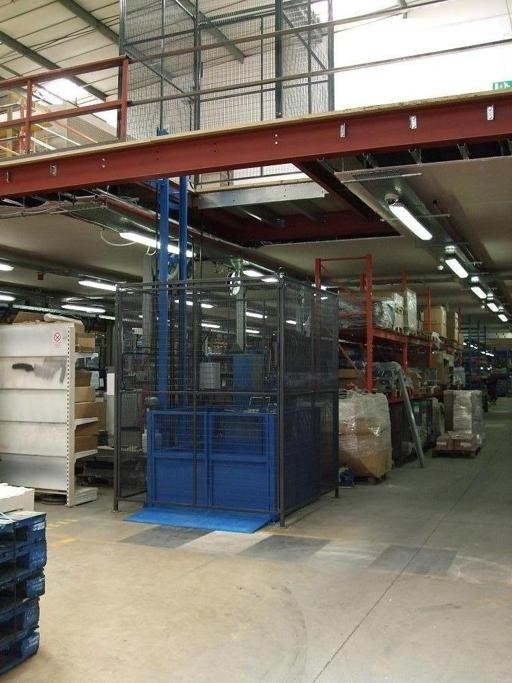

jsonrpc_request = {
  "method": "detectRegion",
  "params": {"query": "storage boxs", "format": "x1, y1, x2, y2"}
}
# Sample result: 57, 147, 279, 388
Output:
72, 330, 99, 452
449, 314, 460, 326
420, 305, 445, 322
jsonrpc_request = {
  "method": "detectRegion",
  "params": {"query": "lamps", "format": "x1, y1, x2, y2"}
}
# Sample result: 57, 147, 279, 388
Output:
118, 228, 198, 259
0, 260, 312, 343
469, 284, 487, 299
487, 302, 507, 324
386, 195, 434, 243
442, 253, 470, 279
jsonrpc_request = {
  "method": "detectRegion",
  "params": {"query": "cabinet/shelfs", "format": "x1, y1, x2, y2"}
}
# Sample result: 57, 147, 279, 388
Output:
318, 319, 512, 476
0, 318, 100, 508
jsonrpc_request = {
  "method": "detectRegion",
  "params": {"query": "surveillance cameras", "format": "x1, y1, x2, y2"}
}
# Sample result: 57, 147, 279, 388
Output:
385, 194, 399, 205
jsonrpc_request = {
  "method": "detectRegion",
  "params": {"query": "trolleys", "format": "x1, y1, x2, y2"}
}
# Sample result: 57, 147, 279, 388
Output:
354, 459, 395, 484
433, 390, 487, 457
